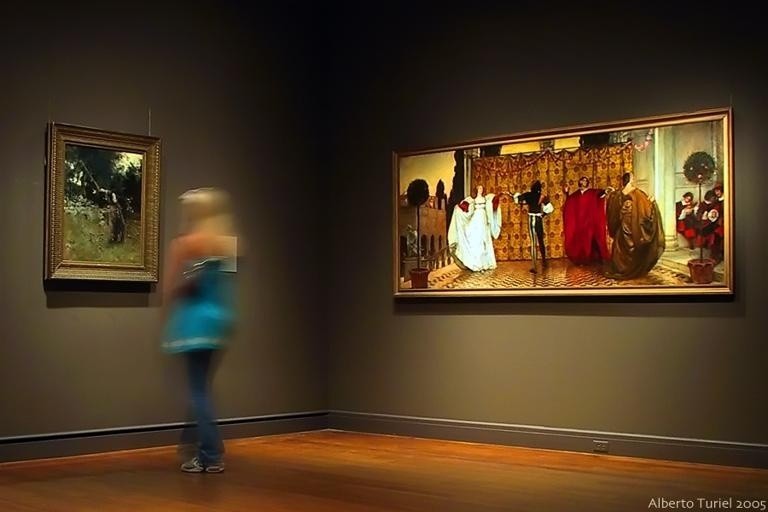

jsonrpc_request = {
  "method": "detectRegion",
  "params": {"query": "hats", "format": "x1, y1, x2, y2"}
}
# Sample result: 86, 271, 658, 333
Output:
712, 183, 723, 191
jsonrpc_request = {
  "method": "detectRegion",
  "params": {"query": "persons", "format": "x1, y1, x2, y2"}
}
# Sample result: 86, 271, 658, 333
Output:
109, 192, 124, 240
560, 176, 613, 266
507, 180, 554, 275
448, 184, 502, 273
704, 185, 724, 260
694, 191, 720, 246
151, 183, 243, 476
675, 192, 700, 252
602, 171, 666, 280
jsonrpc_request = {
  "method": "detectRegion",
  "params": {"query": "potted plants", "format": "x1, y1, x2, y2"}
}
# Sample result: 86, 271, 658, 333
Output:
683, 152, 720, 285
405, 177, 468, 289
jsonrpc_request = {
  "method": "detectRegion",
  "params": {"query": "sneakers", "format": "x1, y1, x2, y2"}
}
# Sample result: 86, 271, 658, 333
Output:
180, 455, 226, 474
528, 267, 538, 274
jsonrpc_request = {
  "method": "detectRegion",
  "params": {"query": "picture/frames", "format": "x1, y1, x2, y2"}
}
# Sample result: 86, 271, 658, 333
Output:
43, 119, 163, 285
391, 106, 737, 298
